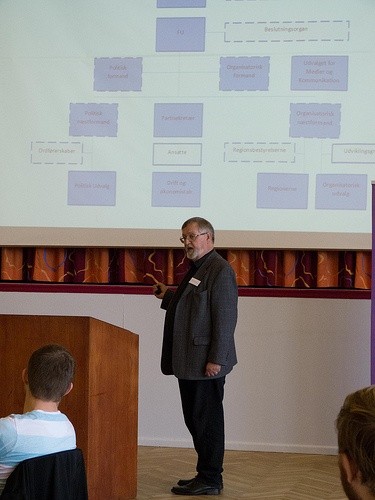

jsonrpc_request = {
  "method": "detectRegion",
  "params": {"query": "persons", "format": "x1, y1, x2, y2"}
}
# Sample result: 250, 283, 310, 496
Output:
153, 216, 238, 496
0, 344, 76, 500
336, 384, 375, 500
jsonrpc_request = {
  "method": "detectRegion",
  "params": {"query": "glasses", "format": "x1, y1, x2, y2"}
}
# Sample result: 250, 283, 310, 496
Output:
179, 232, 210, 243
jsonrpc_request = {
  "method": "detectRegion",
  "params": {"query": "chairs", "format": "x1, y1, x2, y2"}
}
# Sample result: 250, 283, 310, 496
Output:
0, 448, 89, 500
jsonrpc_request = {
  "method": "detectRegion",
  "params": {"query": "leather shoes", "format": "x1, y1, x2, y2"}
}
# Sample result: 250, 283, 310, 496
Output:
171, 477, 221, 494
177, 475, 224, 489
